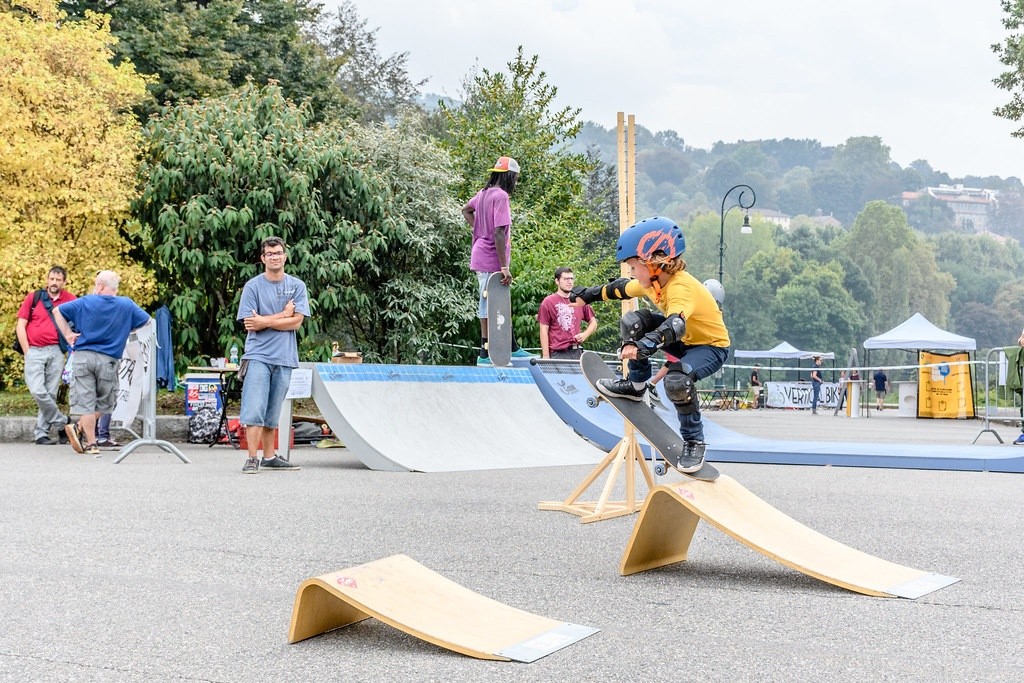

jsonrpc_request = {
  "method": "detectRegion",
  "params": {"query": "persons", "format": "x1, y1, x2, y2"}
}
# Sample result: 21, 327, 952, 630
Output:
751, 363, 762, 408
850, 370, 862, 390
16, 266, 77, 444
1013, 330, 1024, 445
838, 370, 848, 410
873, 370, 887, 410
810, 358, 823, 414
461, 156, 540, 367
568, 216, 730, 474
536, 267, 597, 359
236, 236, 311, 473
210, 385, 216, 393
52, 271, 151, 454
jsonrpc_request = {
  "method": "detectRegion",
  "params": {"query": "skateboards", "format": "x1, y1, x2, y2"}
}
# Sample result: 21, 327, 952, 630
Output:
579, 351, 722, 482
482, 272, 513, 367
292, 414, 329, 430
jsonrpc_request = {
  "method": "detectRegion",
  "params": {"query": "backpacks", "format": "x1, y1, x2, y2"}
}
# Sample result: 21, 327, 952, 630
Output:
187, 401, 227, 444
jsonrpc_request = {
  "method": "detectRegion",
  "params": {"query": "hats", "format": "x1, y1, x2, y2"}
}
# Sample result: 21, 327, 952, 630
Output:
486, 156, 520, 173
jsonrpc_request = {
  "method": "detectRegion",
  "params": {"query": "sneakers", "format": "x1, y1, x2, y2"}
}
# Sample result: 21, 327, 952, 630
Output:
596, 373, 647, 402
510, 347, 540, 359
260, 454, 301, 470
242, 457, 259, 474
1013, 433, 1024, 445
477, 355, 513, 367
677, 440, 710, 473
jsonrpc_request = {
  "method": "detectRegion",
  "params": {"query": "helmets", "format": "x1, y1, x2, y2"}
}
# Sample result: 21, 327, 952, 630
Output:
616, 216, 686, 263
702, 279, 726, 305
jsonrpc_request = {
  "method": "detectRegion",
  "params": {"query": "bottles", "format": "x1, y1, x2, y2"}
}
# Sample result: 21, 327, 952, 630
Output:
230, 345, 238, 363
737, 381, 740, 389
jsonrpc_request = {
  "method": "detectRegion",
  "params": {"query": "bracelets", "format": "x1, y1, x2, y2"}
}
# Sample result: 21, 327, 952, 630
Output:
501, 267, 507, 270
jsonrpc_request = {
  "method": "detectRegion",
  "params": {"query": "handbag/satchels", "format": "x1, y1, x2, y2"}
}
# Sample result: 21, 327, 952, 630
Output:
13, 339, 24, 355
57, 334, 69, 354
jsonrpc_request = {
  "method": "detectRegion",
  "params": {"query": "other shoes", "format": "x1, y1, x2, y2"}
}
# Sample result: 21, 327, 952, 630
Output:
96, 440, 120, 451
58, 416, 71, 444
83, 443, 99, 454
648, 382, 660, 401
64, 421, 85, 453
36, 436, 57, 445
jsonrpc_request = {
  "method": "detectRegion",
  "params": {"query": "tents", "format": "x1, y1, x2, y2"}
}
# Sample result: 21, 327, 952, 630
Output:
862, 312, 978, 420
733, 341, 836, 409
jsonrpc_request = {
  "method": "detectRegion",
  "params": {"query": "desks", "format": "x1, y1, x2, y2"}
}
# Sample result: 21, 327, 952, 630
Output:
716, 390, 751, 410
892, 380, 918, 418
695, 389, 717, 411
844, 381, 869, 417
188, 365, 242, 450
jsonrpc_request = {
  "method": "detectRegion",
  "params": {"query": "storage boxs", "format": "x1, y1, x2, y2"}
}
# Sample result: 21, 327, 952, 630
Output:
236, 425, 295, 450
218, 415, 240, 442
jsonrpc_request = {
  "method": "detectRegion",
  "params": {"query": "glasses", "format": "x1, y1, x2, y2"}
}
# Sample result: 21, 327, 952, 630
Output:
263, 251, 285, 258
